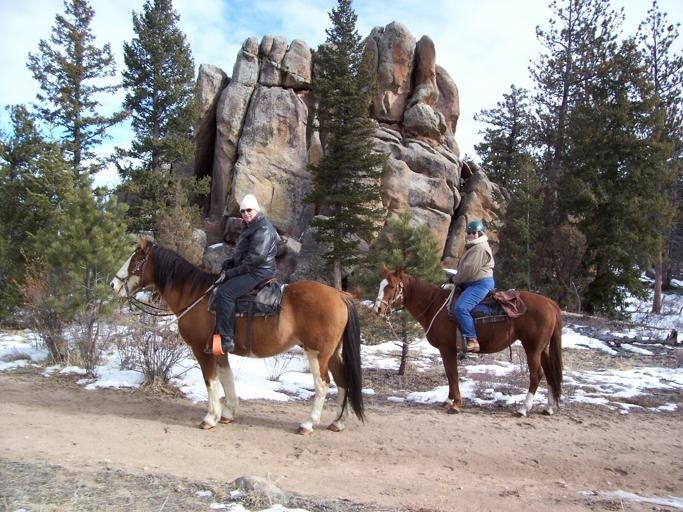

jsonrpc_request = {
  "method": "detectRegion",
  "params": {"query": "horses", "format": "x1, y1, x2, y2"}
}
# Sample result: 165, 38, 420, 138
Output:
109, 233, 368, 436
372, 261, 564, 417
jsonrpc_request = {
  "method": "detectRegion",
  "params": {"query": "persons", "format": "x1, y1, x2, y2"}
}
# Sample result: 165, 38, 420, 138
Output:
202, 191, 284, 355
442, 218, 498, 355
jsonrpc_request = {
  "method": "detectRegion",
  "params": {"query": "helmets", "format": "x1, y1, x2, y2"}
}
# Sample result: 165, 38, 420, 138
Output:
463, 220, 484, 233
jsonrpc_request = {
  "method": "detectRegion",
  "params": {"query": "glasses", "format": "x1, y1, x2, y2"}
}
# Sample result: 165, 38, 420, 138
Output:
466, 231, 477, 236
240, 208, 253, 213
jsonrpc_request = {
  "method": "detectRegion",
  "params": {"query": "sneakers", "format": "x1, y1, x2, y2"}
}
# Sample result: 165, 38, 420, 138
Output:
202, 337, 234, 354
463, 338, 481, 354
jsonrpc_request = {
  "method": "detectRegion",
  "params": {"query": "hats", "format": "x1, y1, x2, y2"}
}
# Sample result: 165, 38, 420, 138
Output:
238, 193, 261, 213
